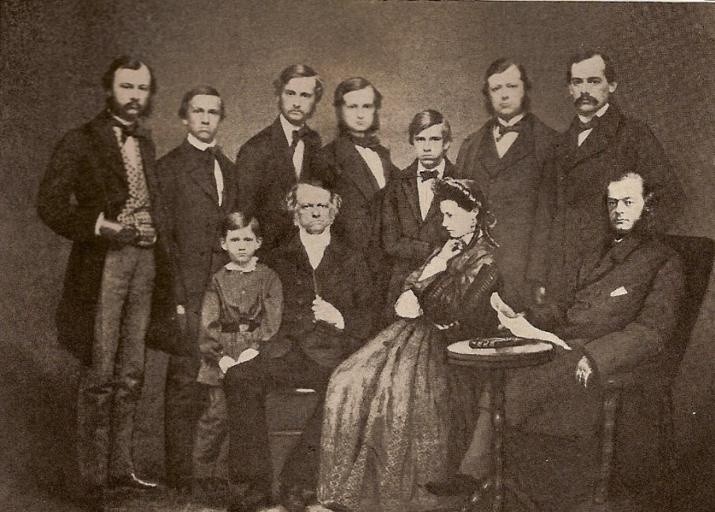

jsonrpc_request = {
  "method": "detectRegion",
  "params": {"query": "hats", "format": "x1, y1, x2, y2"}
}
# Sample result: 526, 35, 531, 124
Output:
431, 176, 490, 216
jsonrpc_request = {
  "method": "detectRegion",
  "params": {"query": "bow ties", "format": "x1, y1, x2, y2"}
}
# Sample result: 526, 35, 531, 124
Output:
496, 123, 526, 134
293, 126, 311, 141
200, 145, 226, 171
356, 135, 381, 150
117, 124, 147, 144
576, 114, 601, 130
420, 167, 441, 181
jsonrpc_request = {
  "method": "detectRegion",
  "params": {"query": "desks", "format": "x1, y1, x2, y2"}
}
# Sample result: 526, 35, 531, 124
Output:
447, 339, 554, 512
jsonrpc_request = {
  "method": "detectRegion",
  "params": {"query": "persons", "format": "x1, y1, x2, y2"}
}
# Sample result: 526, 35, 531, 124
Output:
150, 85, 239, 459
382, 109, 471, 329
36, 56, 161, 512
425, 167, 685, 512
231, 63, 326, 269
322, 76, 403, 328
305, 177, 509, 512
190, 211, 285, 499
524, 49, 688, 315
227, 167, 375, 511
455, 56, 561, 314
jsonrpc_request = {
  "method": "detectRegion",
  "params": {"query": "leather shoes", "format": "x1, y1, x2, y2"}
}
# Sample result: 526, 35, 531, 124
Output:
112, 470, 163, 494
226, 482, 273, 511
275, 475, 312, 512
79, 474, 114, 512
422, 473, 484, 498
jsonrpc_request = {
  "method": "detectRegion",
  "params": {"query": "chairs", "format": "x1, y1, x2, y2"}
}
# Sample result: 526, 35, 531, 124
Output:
261, 217, 371, 437
521, 232, 715, 511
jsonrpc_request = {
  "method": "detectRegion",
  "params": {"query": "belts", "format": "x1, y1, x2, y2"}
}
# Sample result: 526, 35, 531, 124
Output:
222, 320, 266, 335
